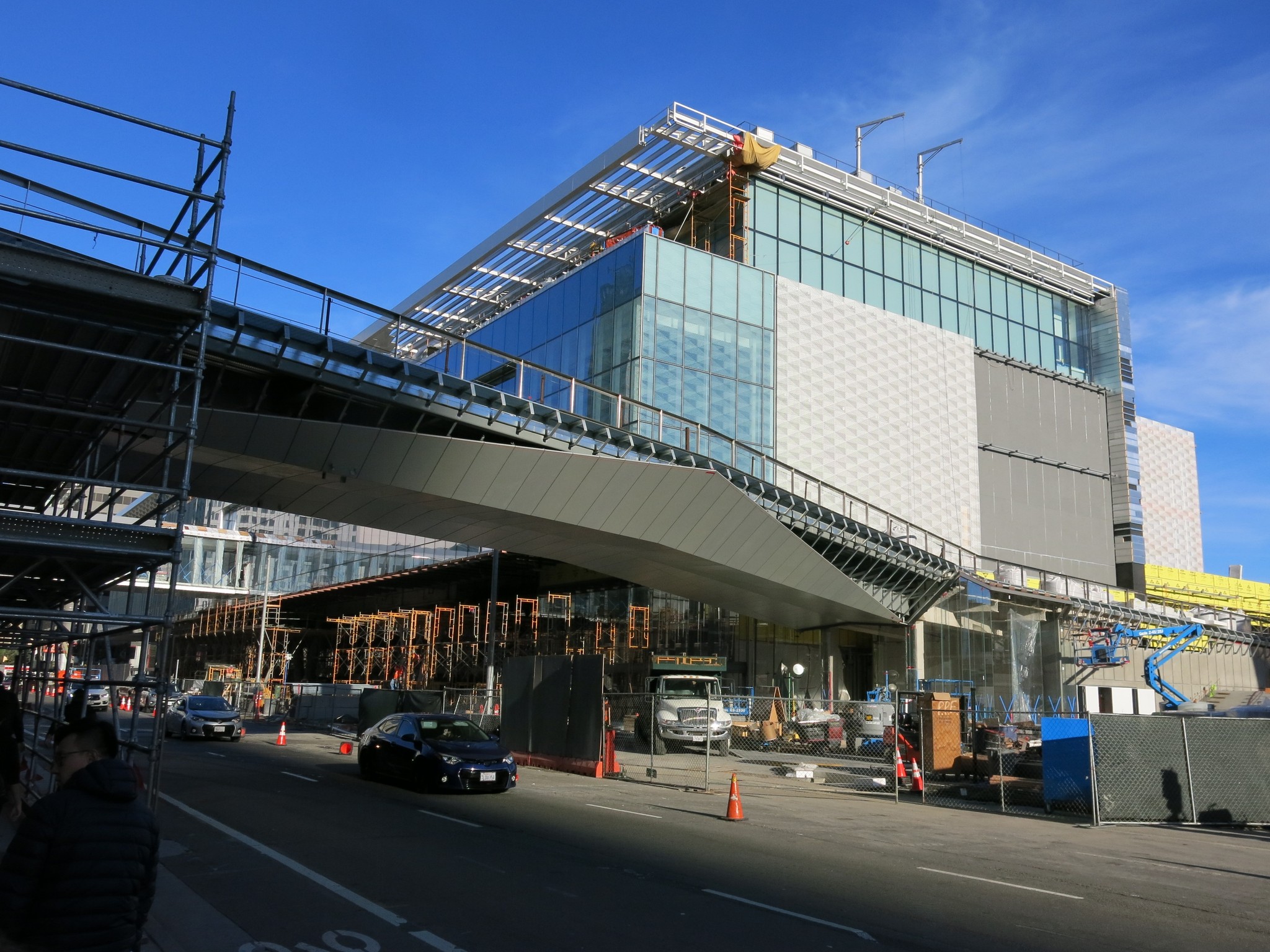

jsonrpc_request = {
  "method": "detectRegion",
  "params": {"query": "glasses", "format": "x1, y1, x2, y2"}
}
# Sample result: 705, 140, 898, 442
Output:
55, 750, 94, 768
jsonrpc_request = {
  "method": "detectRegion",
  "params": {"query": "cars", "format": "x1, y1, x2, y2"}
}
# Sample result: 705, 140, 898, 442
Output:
356, 712, 518, 796
165, 695, 243, 743
0, 665, 110, 712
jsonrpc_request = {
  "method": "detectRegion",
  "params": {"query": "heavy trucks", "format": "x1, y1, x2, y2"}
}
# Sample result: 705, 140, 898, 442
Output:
634, 651, 733, 757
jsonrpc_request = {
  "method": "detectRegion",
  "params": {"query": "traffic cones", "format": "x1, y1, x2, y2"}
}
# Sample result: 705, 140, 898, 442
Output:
119, 696, 126, 710
273, 721, 287, 746
718, 774, 749, 821
893, 746, 907, 778
909, 759, 928, 792
338, 741, 354, 755
124, 697, 132, 711
151, 705, 156, 717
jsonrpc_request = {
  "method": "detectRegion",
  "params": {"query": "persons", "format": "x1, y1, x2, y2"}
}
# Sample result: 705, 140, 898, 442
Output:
0, 718, 159, 952
226, 665, 234, 678
390, 652, 421, 690
0, 671, 25, 822
253, 685, 263, 714
44, 689, 97, 751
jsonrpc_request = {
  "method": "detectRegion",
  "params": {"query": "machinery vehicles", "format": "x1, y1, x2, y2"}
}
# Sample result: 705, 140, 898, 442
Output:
1113, 620, 1270, 720
